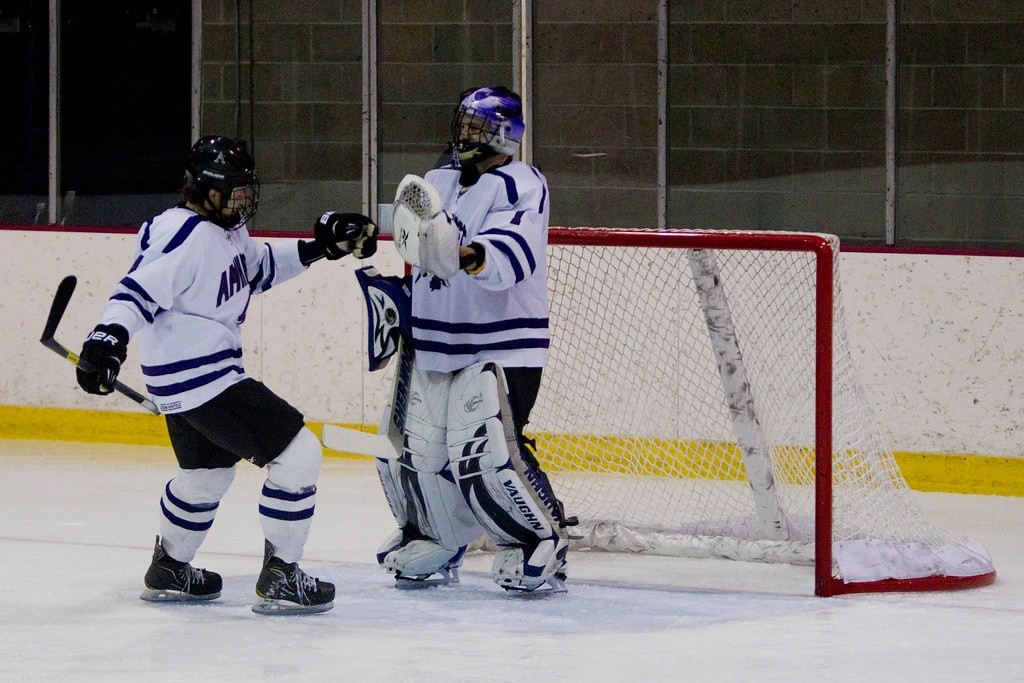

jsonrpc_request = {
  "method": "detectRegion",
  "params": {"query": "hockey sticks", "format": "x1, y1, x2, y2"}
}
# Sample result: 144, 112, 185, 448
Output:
319, 261, 413, 460
39, 275, 163, 416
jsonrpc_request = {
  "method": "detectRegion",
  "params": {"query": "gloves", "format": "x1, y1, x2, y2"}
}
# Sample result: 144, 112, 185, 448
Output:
76, 323, 129, 395
314, 212, 379, 260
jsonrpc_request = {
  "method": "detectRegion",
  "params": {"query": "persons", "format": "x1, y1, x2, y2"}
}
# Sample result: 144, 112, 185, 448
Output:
375, 86, 568, 589
76, 134, 380, 605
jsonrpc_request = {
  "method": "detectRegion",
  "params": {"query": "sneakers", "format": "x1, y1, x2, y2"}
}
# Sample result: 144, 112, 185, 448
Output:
140, 534, 223, 602
499, 559, 568, 600
251, 538, 336, 615
394, 544, 468, 589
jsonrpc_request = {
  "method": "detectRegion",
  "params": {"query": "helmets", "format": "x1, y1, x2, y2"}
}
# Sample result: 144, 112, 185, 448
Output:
185, 135, 260, 232
450, 86, 525, 170
451, 88, 481, 111
